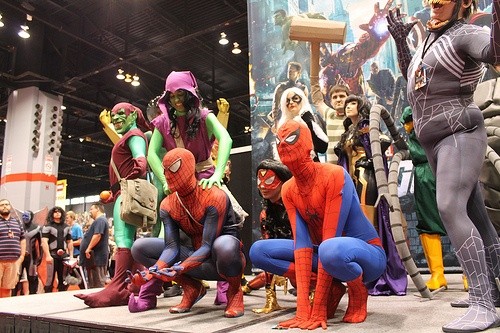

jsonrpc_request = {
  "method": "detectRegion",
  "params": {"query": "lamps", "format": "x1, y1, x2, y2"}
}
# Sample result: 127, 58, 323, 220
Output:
231, 41, 242, 55
16, 19, 32, 40
116, 65, 125, 80
125, 67, 132, 83
131, 69, 141, 87
219, 30, 230, 46
0, 11, 5, 28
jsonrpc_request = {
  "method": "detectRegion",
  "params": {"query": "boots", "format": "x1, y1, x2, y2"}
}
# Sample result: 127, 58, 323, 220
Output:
419, 231, 450, 292
462, 273, 470, 292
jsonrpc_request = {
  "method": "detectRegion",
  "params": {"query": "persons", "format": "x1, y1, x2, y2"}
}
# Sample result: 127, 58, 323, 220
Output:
387, 1, 500, 332
0, 198, 116, 298
370, 97, 499, 302
247, 120, 387, 330
334, 94, 408, 296
273, 86, 329, 162
243, 158, 315, 315
73, 69, 248, 318
289, 18, 350, 165
248, 1, 397, 121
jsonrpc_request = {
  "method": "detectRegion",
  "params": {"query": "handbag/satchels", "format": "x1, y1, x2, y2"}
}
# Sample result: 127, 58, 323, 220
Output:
119, 173, 159, 232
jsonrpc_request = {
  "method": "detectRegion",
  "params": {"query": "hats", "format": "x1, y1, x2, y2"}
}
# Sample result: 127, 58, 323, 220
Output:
157, 70, 205, 106
